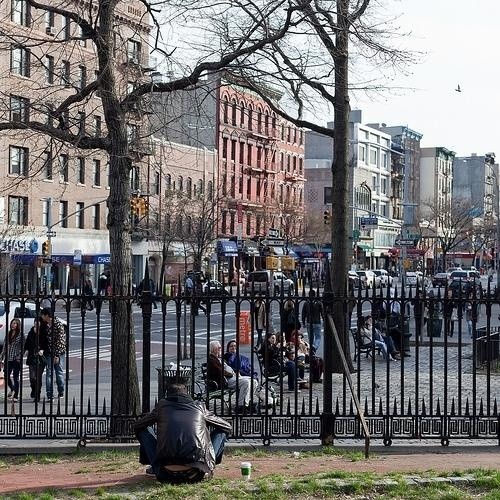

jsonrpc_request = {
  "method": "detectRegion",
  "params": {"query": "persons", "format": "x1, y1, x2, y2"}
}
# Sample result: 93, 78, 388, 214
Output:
131, 278, 157, 309
83, 269, 112, 312
0, 318, 26, 402
183, 273, 207, 316
223, 340, 258, 381
38, 306, 65, 402
133, 383, 233, 484
209, 341, 258, 414
350, 261, 493, 360
25, 316, 46, 402
253, 268, 325, 392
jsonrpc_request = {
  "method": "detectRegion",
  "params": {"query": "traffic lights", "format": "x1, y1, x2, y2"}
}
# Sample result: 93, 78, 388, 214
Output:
140, 198, 150, 217
323, 206, 332, 228
131, 198, 138, 215
41, 239, 52, 258
262, 256, 279, 270
280, 257, 299, 272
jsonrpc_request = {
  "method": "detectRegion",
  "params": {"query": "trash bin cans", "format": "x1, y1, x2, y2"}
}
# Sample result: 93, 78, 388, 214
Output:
156, 365, 194, 407
424, 316, 443, 337
386, 315, 410, 352
166, 284, 179, 298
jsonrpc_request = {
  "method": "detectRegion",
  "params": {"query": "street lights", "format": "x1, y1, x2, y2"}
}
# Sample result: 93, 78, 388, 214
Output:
460, 157, 500, 291
349, 139, 409, 299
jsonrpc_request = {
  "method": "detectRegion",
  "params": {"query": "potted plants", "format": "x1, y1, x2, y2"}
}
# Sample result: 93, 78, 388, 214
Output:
425, 294, 443, 338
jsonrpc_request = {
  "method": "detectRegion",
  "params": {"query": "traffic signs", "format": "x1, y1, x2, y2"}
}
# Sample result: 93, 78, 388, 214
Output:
259, 237, 284, 247
269, 228, 280, 238
45, 231, 56, 238
395, 238, 415, 247
359, 216, 378, 230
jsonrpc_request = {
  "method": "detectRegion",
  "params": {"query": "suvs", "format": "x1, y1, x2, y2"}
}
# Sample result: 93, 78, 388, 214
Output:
243, 270, 296, 298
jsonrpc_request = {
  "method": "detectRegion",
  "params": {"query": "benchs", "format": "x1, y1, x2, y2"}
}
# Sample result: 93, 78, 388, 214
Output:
254, 338, 311, 391
197, 360, 239, 415
349, 327, 385, 362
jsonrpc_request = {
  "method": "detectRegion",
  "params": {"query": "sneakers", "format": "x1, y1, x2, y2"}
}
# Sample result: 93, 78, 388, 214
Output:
146, 466, 156, 476
235, 402, 257, 414
8, 390, 65, 402
448, 332, 454, 337
287, 377, 324, 393
387, 351, 411, 361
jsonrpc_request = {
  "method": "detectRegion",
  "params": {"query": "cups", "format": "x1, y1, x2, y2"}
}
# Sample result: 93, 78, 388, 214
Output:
240, 462, 252, 479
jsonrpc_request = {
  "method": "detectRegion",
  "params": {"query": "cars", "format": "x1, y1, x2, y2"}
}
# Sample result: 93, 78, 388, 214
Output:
0, 300, 70, 353
347, 264, 484, 292
186, 269, 231, 303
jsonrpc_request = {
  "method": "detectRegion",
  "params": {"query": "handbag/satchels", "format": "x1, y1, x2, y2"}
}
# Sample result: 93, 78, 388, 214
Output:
451, 308, 459, 321
0, 367, 5, 386
